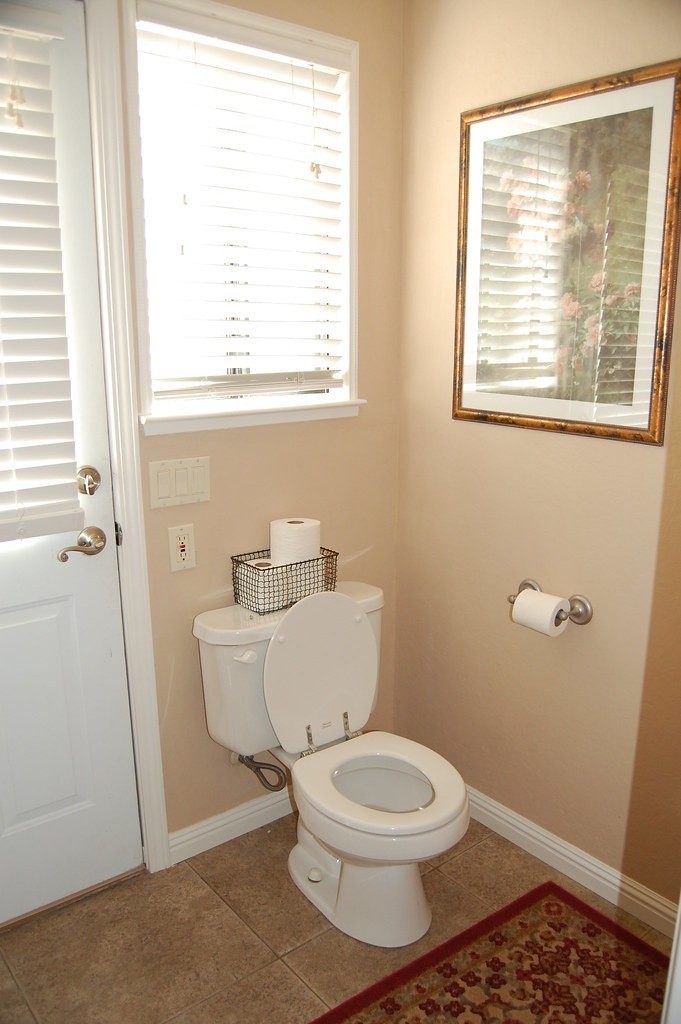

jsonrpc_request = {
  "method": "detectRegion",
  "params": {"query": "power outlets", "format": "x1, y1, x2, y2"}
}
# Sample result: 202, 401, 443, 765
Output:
168, 524, 196, 573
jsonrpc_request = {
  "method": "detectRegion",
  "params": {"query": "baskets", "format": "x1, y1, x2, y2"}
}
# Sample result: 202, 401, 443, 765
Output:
232, 545, 339, 614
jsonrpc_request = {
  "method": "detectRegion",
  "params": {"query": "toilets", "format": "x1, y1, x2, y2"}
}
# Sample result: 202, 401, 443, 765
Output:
191, 572, 471, 951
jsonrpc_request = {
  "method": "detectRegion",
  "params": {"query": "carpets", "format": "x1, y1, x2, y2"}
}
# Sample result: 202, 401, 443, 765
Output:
307, 880, 670, 1024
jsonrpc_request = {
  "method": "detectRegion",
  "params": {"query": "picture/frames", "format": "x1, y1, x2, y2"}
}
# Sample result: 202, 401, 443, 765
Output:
450, 57, 681, 448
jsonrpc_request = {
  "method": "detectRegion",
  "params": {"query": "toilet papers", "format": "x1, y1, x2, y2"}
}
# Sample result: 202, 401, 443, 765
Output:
511, 588, 571, 638
235, 516, 327, 614
